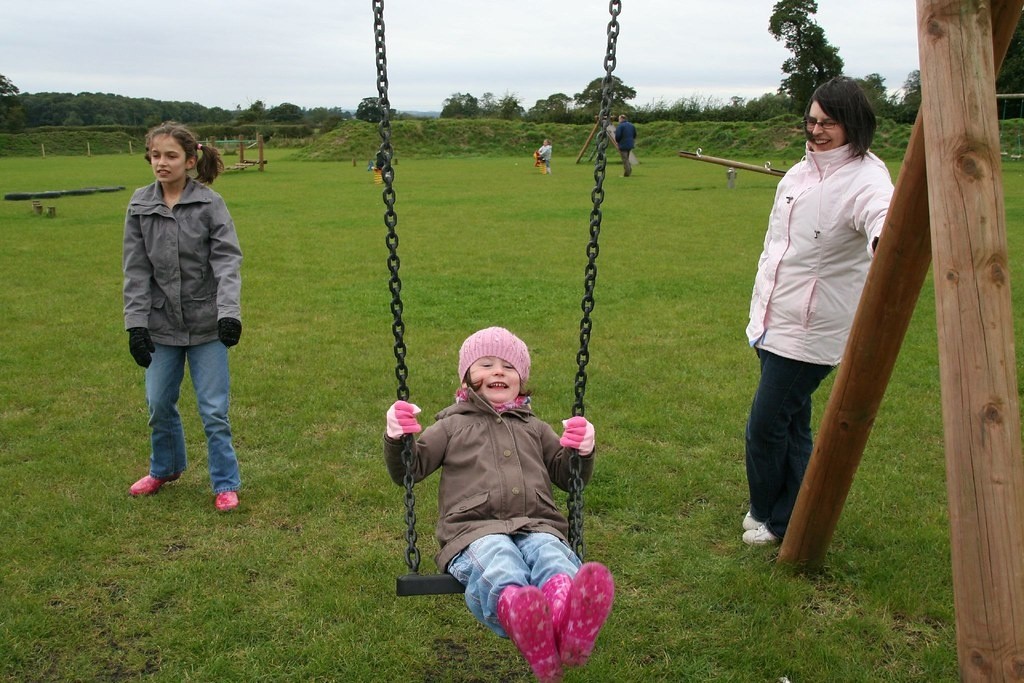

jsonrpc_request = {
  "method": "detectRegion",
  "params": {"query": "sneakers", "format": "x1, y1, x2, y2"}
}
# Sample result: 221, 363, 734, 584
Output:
743, 509, 765, 530
742, 522, 780, 545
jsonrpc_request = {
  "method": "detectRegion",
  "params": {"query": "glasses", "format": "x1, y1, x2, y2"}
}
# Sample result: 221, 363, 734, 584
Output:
801, 117, 839, 129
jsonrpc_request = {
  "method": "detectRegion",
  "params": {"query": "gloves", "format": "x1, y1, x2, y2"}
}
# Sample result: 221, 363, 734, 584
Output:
560, 416, 595, 456
387, 400, 422, 440
217, 317, 242, 347
129, 326, 155, 368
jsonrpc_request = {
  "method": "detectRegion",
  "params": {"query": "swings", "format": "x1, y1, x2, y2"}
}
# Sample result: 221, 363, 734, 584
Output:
371, 0, 623, 597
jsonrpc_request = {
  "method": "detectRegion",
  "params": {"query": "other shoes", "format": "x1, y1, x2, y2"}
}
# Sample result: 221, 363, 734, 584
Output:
129, 469, 181, 497
215, 492, 238, 511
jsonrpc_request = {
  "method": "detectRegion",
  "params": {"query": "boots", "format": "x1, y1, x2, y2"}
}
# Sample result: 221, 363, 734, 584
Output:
542, 561, 614, 666
497, 583, 564, 683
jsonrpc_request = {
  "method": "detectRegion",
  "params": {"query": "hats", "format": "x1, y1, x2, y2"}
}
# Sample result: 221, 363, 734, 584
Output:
458, 327, 531, 387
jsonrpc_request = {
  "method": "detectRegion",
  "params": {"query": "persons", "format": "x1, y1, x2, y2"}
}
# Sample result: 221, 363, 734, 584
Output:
742, 77, 895, 544
383, 327, 615, 683
538, 139, 552, 174
615, 114, 637, 177
122, 125, 243, 511
374, 149, 389, 184
